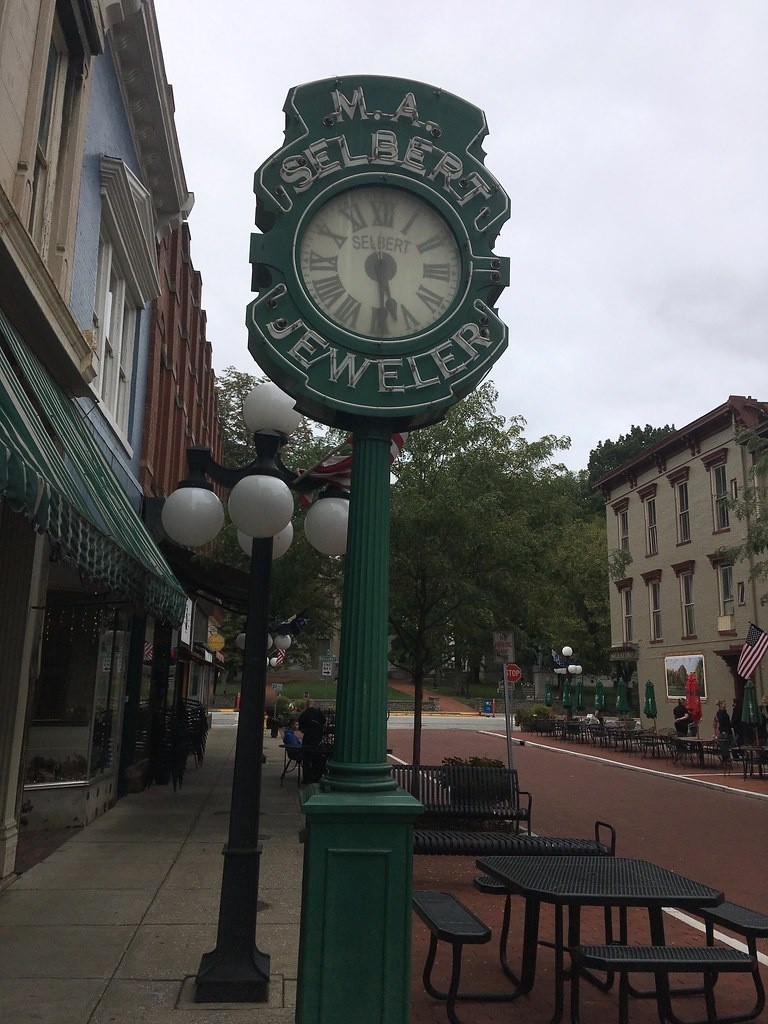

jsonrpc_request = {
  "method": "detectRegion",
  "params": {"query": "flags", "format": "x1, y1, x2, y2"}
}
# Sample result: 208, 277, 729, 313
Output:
276, 649, 285, 666
296, 431, 408, 506
275, 612, 309, 635
549, 647, 566, 674
736, 623, 768, 679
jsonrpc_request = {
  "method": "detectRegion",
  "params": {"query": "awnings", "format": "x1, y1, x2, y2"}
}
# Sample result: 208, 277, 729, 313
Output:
0, 308, 189, 631
163, 542, 277, 621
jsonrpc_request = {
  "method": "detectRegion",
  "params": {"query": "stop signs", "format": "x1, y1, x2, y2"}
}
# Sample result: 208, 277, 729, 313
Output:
505, 664, 521, 682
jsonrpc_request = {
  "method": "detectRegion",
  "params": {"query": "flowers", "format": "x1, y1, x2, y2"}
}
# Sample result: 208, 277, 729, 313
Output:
319, 734, 335, 745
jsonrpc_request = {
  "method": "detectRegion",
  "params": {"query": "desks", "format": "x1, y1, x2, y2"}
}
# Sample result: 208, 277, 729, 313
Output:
476, 857, 726, 1017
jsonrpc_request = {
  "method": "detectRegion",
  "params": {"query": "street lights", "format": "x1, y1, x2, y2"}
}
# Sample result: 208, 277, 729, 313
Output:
154, 381, 349, 1007
561, 646, 583, 720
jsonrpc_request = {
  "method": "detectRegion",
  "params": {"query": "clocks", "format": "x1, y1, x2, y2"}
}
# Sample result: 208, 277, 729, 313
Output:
249, 74, 512, 430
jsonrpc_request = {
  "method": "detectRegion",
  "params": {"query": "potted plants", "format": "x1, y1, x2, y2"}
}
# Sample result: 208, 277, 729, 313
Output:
444, 757, 507, 806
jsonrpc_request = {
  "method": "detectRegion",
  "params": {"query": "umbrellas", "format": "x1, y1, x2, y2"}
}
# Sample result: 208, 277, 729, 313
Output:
615, 680, 631, 728
643, 681, 657, 728
544, 683, 552, 707
684, 674, 702, 739
741, 681, 762, 745
563, 681, 607, 722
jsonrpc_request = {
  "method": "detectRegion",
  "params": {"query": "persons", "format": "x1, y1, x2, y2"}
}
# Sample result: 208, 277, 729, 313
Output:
758, 696, 768, 762
715, 699, 732, 762
673, 698, 698, 753
298, 699, 327, 787
586, 710, 603, 724
284, 719, 303, 767
730, 698, 745, 757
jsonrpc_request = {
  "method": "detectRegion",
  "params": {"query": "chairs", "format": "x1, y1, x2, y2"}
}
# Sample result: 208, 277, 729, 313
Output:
154, 698, 209, 768
278, 744, 302, 779
536, 721, 767, 780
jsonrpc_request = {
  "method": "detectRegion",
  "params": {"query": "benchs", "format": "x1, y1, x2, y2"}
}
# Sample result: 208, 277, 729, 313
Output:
393, 765, 532, 835
570, 945, 753, 1024
701, 901, 768, 947
414, 821, 615, 856
411, 890, 491, 1024
476, 876, 517, 895
